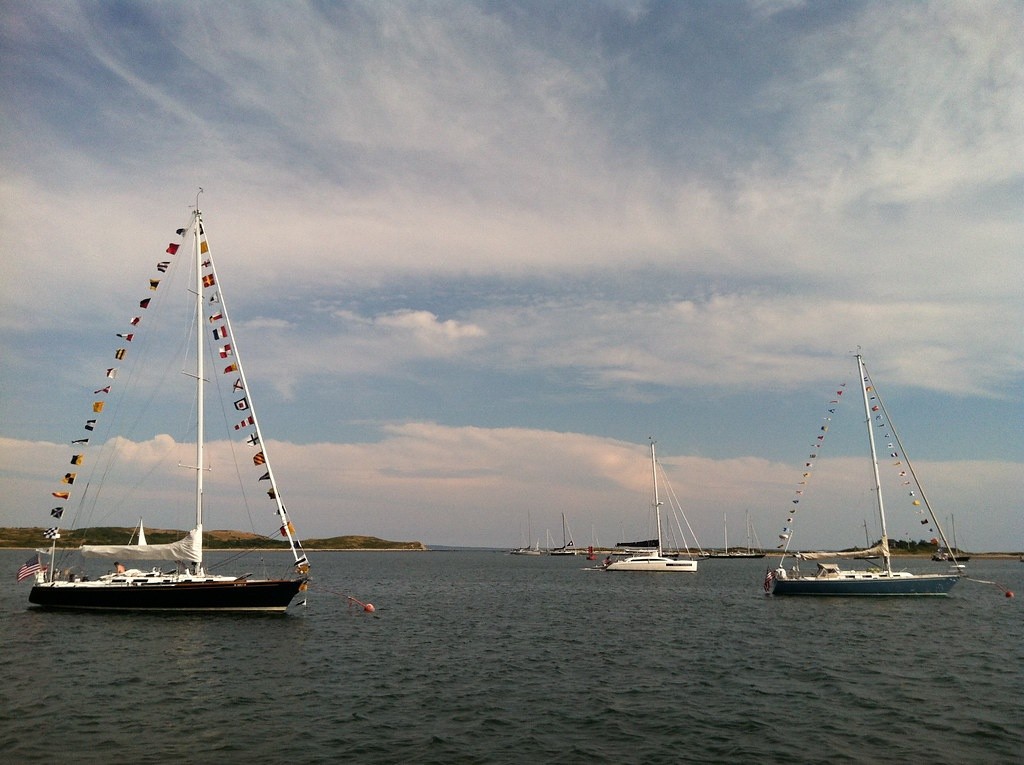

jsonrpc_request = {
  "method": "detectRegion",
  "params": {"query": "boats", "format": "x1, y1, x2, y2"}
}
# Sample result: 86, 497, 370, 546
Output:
698, 551, 710, 556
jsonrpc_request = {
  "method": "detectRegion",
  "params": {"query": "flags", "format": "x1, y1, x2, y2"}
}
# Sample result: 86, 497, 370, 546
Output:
35, 507, 65, 555
885, 434, 910, 486
910, 491, 938, 545
113, 298, 151, 360
812, 383, 846, 447
234, 396, 271, 481
764, 569, 774, 592
775, 453, 815, 549
267, 487, 308, 567
149, 228, 188, 290
16, 554, 42, 582
864, 377, 885, 428
209, 308, 243, 393
85, 367, 118, 432
51, 438, 89, 501
194, 225, 219, 305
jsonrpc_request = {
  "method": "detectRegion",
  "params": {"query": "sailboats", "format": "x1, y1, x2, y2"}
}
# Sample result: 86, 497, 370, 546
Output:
710, 509, 765, 558
510, 506, 541, 556
765, 353, 967, 596
660, 515, 679, 556
580, 522, 601, 554
854, 520, 880, 559
540, 513, 578, 556
611, 520, 633, 555
596, 438, 710, 573
932, 512, 971, 561
29, 207, 313, 618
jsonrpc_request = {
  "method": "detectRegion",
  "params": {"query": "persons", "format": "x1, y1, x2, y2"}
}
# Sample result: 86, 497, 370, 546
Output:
114, 562, 125, 573
